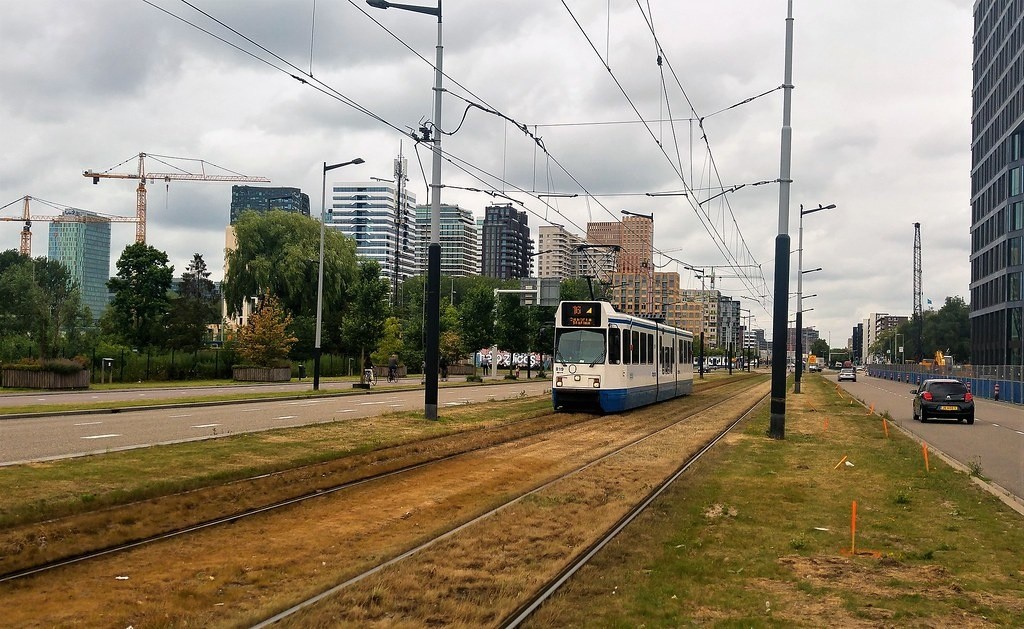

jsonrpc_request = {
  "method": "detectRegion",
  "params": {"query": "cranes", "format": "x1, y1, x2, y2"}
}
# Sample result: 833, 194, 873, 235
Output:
82, 151, 272, 245
0, 192, 141, 258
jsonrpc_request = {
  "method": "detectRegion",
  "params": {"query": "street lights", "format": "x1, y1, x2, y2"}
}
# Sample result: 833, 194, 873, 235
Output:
737, 308, 751, 372
794, 204, 837, 394
620, 209, 654, 314
314, 158, 366, 390
365, 0, 445, 422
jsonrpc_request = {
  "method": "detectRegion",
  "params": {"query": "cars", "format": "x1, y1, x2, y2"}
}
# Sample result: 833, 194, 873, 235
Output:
910, 378, 975, 425
835, 360, 868, 371
698, 364, 711, 373
837, 367, 856, 382
731, 357, 772, 369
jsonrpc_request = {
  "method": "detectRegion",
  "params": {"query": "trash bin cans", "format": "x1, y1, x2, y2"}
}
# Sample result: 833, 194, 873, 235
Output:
103, 358, 113, 371
299, 365, 306, 378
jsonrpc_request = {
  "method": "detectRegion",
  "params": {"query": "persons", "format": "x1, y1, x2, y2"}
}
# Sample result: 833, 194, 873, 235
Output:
481, 355, 490, 377
515, 365, 520, 379
388, 355, 399, 382
363, 356, 375, 383
439, 355, 450, 382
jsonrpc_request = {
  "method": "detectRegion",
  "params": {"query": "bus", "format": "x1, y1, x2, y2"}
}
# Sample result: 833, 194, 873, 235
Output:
550, 243, 695, 416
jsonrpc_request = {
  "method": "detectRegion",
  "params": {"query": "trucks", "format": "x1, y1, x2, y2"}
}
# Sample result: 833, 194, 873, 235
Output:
791, 357, 825, 373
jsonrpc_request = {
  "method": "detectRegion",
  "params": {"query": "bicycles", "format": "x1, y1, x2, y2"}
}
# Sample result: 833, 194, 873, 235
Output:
387, 364, 399, 383
364, 366, 377, 386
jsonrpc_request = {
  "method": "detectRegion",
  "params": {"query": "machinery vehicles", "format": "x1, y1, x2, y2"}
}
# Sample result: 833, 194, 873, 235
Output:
920, 350, 953, 376
905, 222, 923, 364
